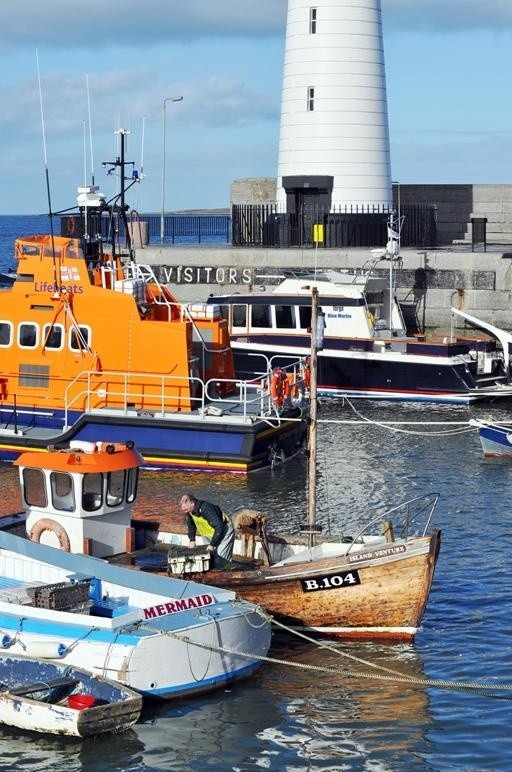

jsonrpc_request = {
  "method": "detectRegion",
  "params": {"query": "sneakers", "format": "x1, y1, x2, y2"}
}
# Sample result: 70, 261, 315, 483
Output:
210, 567, 223, 572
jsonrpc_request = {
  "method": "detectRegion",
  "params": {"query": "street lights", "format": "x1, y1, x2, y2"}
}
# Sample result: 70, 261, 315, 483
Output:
160, 95, 184, 244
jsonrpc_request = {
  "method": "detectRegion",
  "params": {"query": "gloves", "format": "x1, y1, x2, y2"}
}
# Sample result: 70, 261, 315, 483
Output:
187, 541, 196, 549
206, 544, 214, 552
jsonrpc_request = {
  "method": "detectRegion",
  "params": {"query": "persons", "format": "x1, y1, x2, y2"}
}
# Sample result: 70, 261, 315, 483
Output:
179, 495, 235, 570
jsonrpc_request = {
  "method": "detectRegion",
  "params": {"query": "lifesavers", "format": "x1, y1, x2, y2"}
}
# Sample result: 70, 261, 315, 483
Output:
304, 356, 311, 389
28, 518, 70, 552
271, 370, 290, 405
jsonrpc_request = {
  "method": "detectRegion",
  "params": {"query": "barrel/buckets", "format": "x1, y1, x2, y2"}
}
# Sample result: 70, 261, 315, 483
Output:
69, 694, 96, 711
